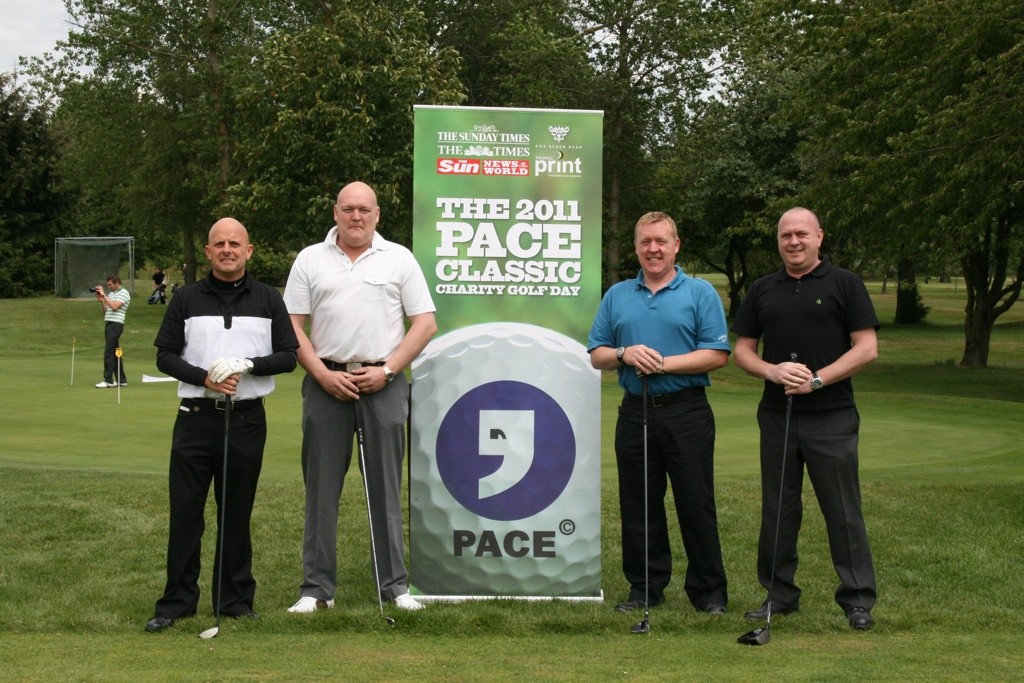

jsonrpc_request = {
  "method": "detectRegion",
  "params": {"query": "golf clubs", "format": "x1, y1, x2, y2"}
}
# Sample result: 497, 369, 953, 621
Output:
736, 352, 799, 645
353, 398, 397, 627
630, 379, 650, 634
197, 394, 231, 640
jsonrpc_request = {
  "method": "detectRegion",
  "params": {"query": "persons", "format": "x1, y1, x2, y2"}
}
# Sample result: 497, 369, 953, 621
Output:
282, 181, 438, 612
145, 218, 300, 633
151, 267, 168, 304
728, 207, 882, 629
95, 274, 130, 388
586, 213, 732, 615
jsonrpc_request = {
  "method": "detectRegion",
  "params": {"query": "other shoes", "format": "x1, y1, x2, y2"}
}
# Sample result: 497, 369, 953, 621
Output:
114, 381, 127, 386
704, 601, 726, 615
95, 381, 117, 387
616, 600, 642, 613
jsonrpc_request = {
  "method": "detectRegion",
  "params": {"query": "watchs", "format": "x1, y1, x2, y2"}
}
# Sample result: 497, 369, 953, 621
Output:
245, 359, 254, 373
810, 372, 823, 391
616, 346, 626, 364
101, 294, 105, 298
383, 365, 395, 384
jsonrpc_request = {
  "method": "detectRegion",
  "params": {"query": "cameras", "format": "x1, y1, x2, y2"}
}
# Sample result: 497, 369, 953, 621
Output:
90, 287, 98, 292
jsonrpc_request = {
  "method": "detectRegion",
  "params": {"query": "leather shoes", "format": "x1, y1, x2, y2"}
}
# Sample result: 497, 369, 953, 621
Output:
845, 608, 873, 630
243, 610, 259, 619
144, 616, 174, 635
741, 601, 799, 619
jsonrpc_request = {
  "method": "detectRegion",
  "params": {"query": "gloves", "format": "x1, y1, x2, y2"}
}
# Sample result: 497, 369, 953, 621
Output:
207, 356, 255, 385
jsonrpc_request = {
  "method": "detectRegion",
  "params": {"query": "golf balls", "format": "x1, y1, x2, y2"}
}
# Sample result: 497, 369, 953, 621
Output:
411, 321, 602, 597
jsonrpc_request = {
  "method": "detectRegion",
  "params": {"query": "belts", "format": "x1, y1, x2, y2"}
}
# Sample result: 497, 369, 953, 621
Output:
624, 386, 706, 408
319, 358, 388, 375
193, 397, 263, 411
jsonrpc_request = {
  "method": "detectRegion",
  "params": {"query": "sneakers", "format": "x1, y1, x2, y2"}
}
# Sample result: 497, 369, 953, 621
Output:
394, 593, 428, 612
288, 595, 334, 615
